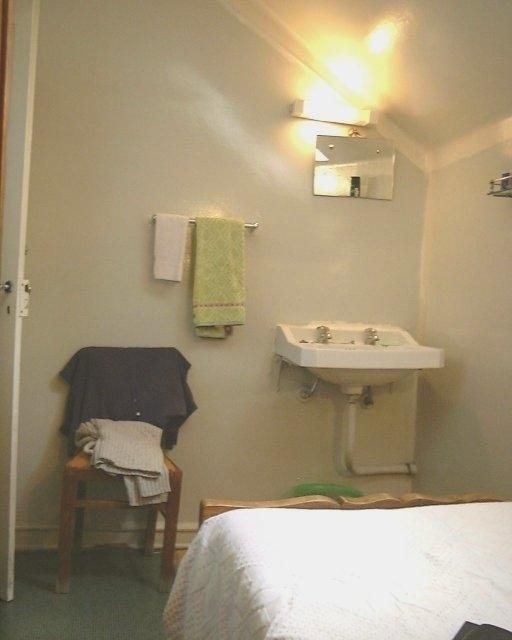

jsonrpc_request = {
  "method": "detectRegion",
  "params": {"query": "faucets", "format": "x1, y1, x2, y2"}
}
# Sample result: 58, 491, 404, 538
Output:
364, 328, 379, 345
316, 326, 332, 343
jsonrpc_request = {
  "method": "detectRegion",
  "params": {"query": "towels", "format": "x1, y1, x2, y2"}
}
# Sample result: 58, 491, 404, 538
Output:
191, 216, 247, 340
151, 212, 186, 282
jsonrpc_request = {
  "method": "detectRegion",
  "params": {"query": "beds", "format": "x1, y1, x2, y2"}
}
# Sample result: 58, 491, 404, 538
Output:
164, 488, 512, 640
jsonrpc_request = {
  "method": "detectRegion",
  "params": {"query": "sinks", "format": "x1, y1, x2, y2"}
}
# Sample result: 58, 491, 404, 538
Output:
272, 324, 446, 396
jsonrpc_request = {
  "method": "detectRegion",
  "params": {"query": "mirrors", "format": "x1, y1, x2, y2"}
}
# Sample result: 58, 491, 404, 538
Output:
311, 135, 398, 202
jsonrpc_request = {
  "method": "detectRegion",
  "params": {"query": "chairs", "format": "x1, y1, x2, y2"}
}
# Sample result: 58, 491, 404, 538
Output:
59, 345, 188, 596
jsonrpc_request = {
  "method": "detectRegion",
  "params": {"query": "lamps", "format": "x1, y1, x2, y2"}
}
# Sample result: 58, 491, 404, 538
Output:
288, 98, 377, 128
